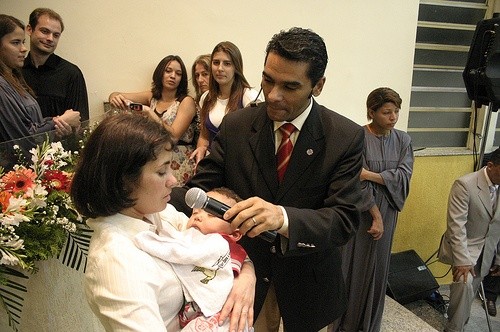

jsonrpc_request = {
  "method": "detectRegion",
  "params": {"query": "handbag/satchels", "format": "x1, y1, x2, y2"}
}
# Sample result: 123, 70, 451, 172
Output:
385, 250, 439, 305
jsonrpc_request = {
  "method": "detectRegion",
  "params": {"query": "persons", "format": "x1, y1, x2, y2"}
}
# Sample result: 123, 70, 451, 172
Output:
20, 8, 90, 152
132, 186, 255, 332
69, 110, 256, 332
0, 14, 81, 169
108, 41, 261, 188
437, 147, 500, 332
326, 87, 415, 332
167, 27, 365, 332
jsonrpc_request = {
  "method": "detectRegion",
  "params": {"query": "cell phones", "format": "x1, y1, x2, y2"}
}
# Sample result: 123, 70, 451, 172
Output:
126, 103, 143, 111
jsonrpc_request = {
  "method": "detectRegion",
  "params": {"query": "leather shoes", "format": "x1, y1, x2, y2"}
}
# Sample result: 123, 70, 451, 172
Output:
487, 300, 496, 316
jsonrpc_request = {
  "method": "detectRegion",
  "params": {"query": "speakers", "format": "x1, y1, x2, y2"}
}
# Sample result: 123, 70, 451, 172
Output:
386, 249, 440, 305
463, 16, 500, 112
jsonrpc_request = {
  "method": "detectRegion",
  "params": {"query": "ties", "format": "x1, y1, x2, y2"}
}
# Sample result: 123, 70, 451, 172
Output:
276, 123, 297, 188
490, 186, 497, 215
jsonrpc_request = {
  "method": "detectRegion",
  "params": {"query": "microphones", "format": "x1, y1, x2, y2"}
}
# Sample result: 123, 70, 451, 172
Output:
184, 187, 277, 243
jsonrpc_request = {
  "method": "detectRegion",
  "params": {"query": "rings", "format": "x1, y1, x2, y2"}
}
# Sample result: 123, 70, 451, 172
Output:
252, 217, 258, 225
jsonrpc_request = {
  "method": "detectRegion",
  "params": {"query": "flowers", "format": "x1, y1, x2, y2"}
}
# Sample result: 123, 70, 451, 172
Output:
0, 114, 104, 332
